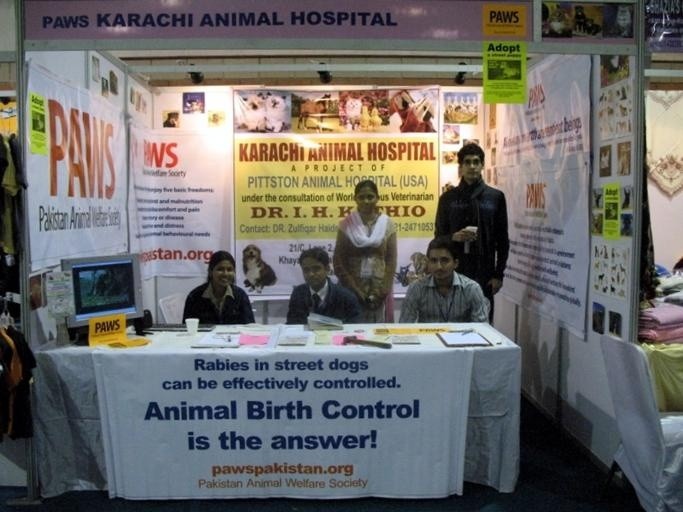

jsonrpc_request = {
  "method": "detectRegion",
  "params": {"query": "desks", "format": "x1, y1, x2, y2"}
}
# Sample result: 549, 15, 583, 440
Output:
31, 321, 520, 498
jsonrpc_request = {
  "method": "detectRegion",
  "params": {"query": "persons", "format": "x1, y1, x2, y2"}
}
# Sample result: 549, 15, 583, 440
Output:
350, 96, 382, 131
397, 236, 491, 323
433, 143, 510, 327
329, 180, 397, 323
180, 251, 255, 325
241, 91, 290, 132
29, 275, 41, 310
285, 246, 364, 324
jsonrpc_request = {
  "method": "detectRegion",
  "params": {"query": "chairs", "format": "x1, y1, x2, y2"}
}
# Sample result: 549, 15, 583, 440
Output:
600, 330, 683, 510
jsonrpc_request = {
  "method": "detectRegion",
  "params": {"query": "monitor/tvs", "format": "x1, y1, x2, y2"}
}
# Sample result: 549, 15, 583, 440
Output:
61, 253, 145, 346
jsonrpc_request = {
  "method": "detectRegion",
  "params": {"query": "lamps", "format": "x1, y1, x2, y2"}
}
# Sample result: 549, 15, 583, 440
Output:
455, 62, 466, 85
187, 63, 205, 85
316, 62, 334, 84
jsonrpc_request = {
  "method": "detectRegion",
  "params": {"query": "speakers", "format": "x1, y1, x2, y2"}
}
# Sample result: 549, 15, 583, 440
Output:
132, 309, 154, 336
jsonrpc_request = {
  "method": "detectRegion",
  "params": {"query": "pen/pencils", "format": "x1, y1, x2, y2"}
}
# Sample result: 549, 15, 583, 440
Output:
461, 330, 472, 336
229, 335, 231, 342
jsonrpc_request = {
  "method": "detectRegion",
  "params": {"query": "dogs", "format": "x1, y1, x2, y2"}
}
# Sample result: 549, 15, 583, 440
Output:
242, 91, 287, 134
343, 94, 366, 132
240, 244, 277, 293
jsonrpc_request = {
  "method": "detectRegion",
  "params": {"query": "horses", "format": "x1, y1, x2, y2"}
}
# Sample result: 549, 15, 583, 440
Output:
297, 94, 331, 133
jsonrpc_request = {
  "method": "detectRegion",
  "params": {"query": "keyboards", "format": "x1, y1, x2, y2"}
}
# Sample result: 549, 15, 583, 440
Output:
133, 323, 216, 332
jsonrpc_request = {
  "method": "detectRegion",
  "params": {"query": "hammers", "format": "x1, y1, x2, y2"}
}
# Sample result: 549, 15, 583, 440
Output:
344, 336, 392, 349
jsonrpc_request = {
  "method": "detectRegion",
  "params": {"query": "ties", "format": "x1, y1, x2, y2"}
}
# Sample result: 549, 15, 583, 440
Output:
310, 293, 322, 314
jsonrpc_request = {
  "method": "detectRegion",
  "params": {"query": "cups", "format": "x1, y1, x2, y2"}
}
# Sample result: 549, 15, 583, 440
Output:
467, 226, 477, 240
185, 318, 199, 334
